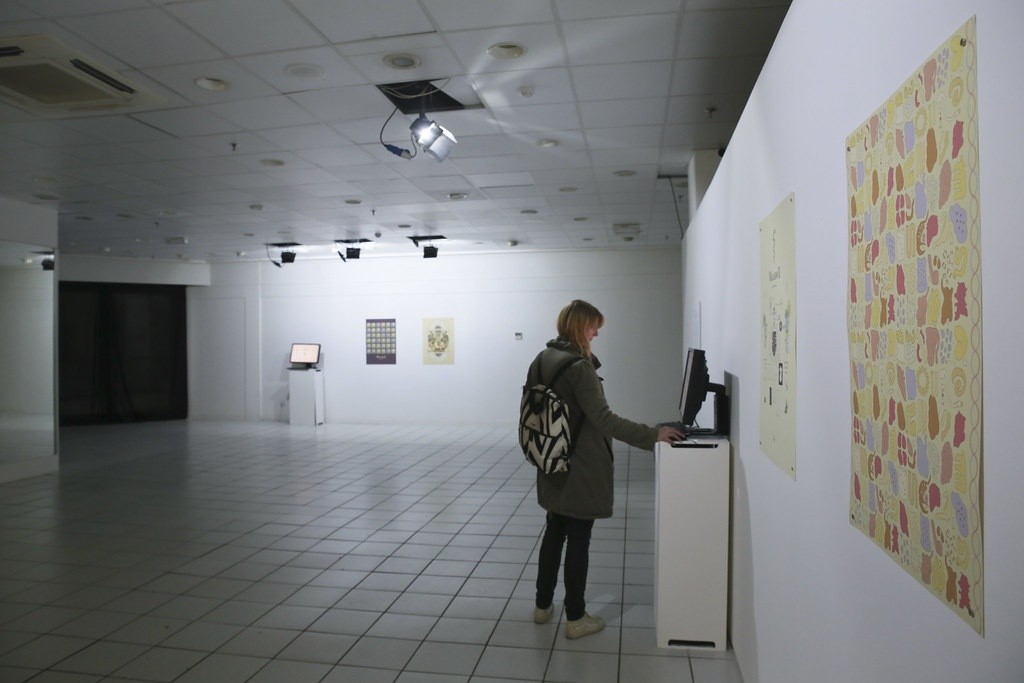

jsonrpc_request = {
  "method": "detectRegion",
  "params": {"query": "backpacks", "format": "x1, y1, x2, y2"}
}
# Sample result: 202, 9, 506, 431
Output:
519, 351, 586, 473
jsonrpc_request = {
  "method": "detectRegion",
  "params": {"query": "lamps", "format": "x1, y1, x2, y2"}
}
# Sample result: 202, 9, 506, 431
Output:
41, 252, 54, 270
410, 91, 458, 162
424, 239, 438, 258
346, 243, 361, 258
281, 244, 296, 262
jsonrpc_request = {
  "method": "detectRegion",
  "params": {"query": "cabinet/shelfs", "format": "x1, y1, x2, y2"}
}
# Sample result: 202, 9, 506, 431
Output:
654, 429, 731, 653
289, 369, 324, 426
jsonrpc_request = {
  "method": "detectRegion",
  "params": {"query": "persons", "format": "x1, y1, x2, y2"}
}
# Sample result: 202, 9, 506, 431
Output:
525, 300, 686, 640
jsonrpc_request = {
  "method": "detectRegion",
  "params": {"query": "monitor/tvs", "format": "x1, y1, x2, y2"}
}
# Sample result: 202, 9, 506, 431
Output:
678, 348, 727, 434
289, 343, 321, 370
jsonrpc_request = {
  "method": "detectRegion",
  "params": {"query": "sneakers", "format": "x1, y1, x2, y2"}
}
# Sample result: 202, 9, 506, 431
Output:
565, 612, 605, 639
533, 603, 555, 624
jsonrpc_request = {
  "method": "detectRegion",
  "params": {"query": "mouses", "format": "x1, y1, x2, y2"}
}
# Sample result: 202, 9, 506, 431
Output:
669, 433, 687, 440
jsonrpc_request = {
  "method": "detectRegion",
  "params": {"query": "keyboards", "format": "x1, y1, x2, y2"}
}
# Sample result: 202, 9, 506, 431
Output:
655, 421, 690, 436
